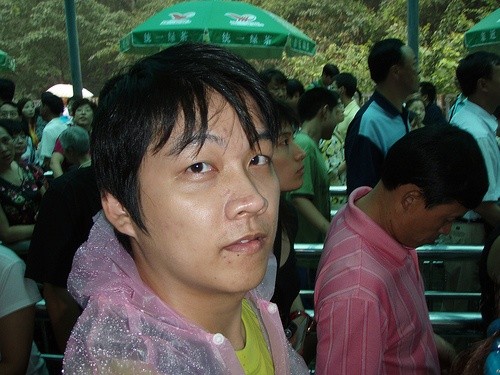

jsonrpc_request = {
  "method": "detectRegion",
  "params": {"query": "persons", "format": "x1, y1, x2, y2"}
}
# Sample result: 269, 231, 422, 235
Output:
0, 38, 500, 375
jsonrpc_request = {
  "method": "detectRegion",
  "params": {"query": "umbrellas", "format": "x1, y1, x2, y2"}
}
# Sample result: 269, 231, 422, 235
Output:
45, 83, 95, 99
462, 6, 500, 54
0, 50, 16, 72
118, 0, 317, 59
